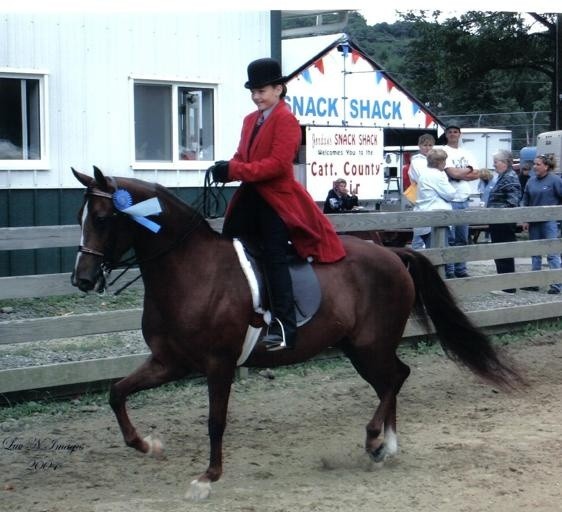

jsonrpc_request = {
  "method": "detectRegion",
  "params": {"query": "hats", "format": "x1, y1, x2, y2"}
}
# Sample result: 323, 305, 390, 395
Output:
244, 57, 288, 90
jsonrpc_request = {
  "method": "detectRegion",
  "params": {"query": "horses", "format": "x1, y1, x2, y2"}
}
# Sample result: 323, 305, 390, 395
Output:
67, 164, 534, 502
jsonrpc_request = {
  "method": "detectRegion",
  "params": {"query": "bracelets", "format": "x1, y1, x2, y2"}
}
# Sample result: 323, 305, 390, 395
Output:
467, 165, 473, 172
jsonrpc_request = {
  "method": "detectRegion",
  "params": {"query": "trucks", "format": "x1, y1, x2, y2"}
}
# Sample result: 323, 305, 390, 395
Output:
380, 128, 562, 244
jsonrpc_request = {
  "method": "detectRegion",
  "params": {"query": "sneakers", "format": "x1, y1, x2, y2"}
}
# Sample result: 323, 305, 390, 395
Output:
448, 272, 471, 281
492, 283, 559, 296
261, 335, 295, 351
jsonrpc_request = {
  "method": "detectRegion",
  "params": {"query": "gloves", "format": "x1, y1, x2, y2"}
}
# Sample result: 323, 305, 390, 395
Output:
211, 159, 232, 183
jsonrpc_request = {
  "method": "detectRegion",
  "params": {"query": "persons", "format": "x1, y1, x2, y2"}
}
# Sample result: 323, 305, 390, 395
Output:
518, 152, 561, 295
323, 177, 360, 214
210, 55, 347, 353
517, 162, 531, 195
486, 148, 523, 296
412, 147, 457, 278
406, 134, 450, 276
440, 126, 482, 279
477, 167, 500, 243
